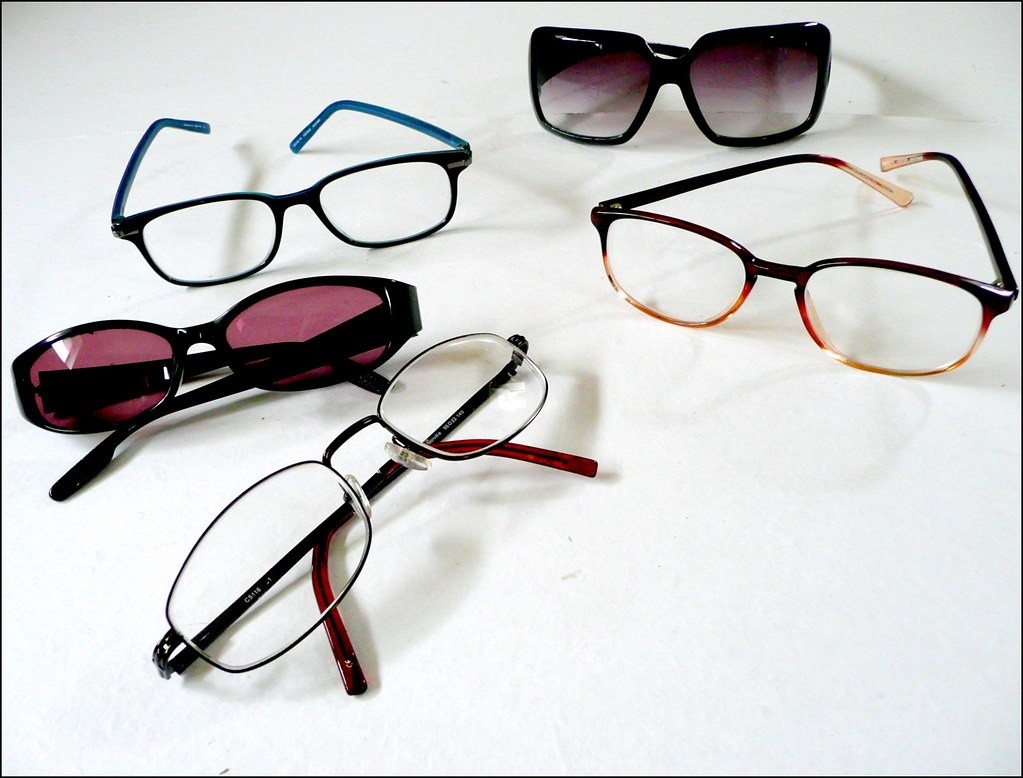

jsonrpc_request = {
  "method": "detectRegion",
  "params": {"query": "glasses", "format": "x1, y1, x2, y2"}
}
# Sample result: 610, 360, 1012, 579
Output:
529, 21, 831, 147
590, 151, 1019, 377
12, 276, 423, 501
110, 100, 473, 287
147, 332, 598, 695
